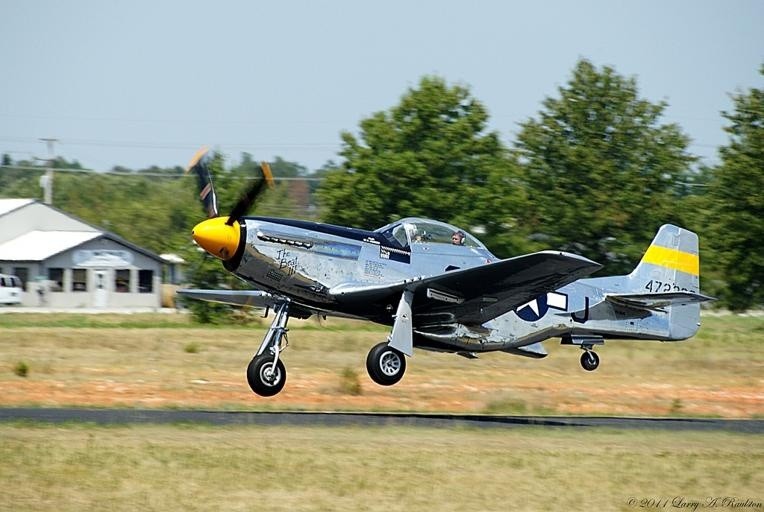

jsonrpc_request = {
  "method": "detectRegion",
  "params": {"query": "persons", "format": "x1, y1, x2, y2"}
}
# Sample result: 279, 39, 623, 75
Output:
412, 234, 424, 242
448, 230, 469, 245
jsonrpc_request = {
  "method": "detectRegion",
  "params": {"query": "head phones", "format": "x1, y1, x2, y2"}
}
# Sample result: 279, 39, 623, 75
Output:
458, 231, 466, 245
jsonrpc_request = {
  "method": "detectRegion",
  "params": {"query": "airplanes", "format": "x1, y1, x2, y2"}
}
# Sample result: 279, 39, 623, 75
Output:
170, 147, 718, 398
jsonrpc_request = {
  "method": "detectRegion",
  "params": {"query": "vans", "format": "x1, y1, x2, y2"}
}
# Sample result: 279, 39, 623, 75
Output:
0, 274, 23, 307
